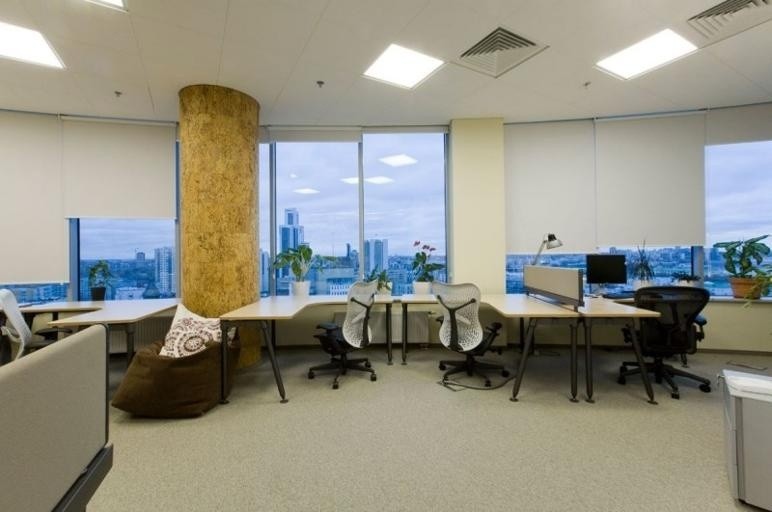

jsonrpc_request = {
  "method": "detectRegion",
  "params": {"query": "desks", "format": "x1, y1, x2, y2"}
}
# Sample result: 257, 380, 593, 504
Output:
529, 286, 692, 404
219, 292, 394, 405
17, 298, 182, 369
400, 291, 579, 403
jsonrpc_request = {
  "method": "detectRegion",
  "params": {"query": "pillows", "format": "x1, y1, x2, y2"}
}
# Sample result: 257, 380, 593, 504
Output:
159, 304, 236, 359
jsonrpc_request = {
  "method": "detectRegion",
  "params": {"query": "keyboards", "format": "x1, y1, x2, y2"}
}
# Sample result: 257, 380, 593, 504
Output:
603, 293, 635, 298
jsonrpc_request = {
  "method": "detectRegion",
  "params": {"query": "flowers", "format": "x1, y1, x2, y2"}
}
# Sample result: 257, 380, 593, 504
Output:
411, 240, 444, 281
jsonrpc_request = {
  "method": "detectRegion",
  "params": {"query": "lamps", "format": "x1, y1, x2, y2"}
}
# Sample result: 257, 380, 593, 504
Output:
532, 233, 563, 266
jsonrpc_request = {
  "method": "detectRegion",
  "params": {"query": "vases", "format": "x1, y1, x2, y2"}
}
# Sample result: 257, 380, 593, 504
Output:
413, 282, 432, 296
264, 244, 336, 296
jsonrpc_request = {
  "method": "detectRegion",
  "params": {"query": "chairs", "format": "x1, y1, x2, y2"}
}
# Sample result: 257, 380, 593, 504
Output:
0, 289, 72, 360
612, 284, 713, 407
307, 277, 382, 389
431, 280, 510, 389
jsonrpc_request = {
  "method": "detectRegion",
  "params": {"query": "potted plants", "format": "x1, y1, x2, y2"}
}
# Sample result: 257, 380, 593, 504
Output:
672, 269, 702, 288
364, 265, 393, 297
714, 232, 772, 299
626, 241, 655, 290
86, 260, 110, 300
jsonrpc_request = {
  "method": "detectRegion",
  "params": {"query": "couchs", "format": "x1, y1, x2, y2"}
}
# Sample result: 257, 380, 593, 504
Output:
111, 328, 244, 420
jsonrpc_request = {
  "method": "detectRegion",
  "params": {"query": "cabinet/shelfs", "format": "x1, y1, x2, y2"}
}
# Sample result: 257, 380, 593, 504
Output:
717, 369, 771, 511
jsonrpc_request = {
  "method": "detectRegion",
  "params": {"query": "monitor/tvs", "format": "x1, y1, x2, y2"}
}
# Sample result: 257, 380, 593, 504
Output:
586, 254, 627, 284
